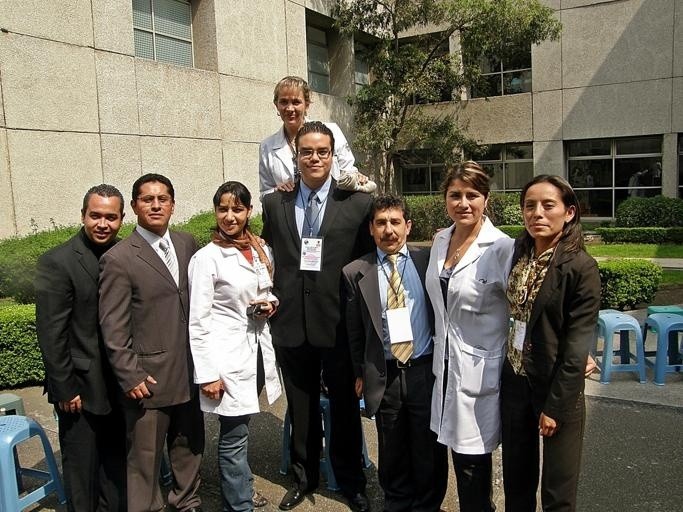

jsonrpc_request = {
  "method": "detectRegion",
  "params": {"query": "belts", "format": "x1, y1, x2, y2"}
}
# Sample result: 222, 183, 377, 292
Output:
388, 360, 419, 370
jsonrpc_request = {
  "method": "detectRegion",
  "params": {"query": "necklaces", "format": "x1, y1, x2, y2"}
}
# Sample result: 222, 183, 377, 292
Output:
451, 226, 480, 260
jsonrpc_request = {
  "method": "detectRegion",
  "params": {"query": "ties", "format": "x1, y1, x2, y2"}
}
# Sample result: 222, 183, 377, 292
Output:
302, 193, 320, 238
385, 253, 414, 364
159, 238, 178, 286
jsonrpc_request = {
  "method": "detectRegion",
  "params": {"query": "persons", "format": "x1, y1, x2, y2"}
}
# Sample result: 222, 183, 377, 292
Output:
186, 180, 284, 512
498, 175, 602, 511
97, 173, 205, 512
339, 196, 448, 512
260, 121, 376, 512
628, 162, 661, 197
424, 160, 517, 512
258, 76, 369, 203
34, 184, 133, 512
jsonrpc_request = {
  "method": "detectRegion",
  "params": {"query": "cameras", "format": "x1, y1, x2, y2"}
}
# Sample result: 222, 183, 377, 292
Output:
246, 303, 269, 316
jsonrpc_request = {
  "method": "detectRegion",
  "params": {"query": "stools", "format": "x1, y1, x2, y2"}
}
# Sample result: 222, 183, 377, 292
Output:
0, 414, 65, 511
641, 313, 683, 385
0, 393, 26, 416
597, 309, 624, 316
596, 312, 646, 385
279, 390, 373, 493
641, 305, 682, 351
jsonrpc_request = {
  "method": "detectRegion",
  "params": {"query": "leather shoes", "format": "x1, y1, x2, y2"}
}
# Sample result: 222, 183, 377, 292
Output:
350, 489, 372, 510
279, 483, 306, 510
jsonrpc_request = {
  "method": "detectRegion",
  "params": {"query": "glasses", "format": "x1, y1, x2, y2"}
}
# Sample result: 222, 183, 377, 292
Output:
298, 149, 331, 161
